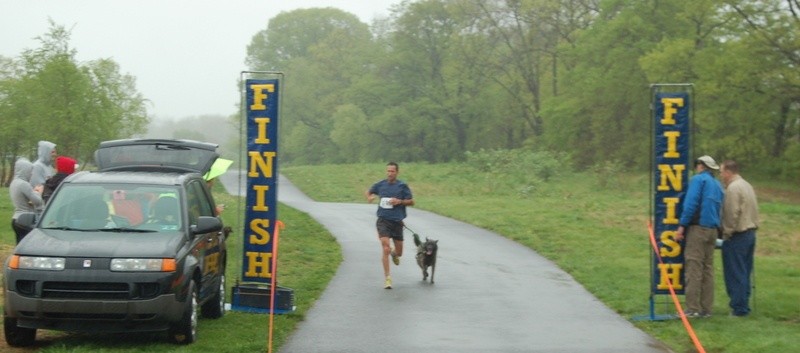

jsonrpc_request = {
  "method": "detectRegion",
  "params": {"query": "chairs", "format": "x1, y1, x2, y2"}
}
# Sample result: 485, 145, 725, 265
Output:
153, 195, 180, 225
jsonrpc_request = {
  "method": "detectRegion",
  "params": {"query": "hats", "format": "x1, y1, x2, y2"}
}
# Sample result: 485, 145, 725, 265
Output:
74, 163, 80, 169
697, 155, 721, 170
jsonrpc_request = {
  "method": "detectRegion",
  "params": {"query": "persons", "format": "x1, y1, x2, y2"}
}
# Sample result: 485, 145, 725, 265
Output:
367, 161, 414, 288
716, 159, 760, 316
674, 155, 725, 319
9, 140, 76, 246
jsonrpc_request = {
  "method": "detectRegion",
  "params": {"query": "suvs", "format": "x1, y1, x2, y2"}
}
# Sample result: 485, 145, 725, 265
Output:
2, 138, 233, 346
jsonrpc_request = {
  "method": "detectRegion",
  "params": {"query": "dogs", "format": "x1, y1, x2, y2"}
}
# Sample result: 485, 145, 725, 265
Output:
413, 234, 438, 283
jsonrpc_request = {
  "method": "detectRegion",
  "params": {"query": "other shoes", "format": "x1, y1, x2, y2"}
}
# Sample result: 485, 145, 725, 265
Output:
384, 276, 393, 289
677, 311, 703, 319
390, 245, 400, 265
